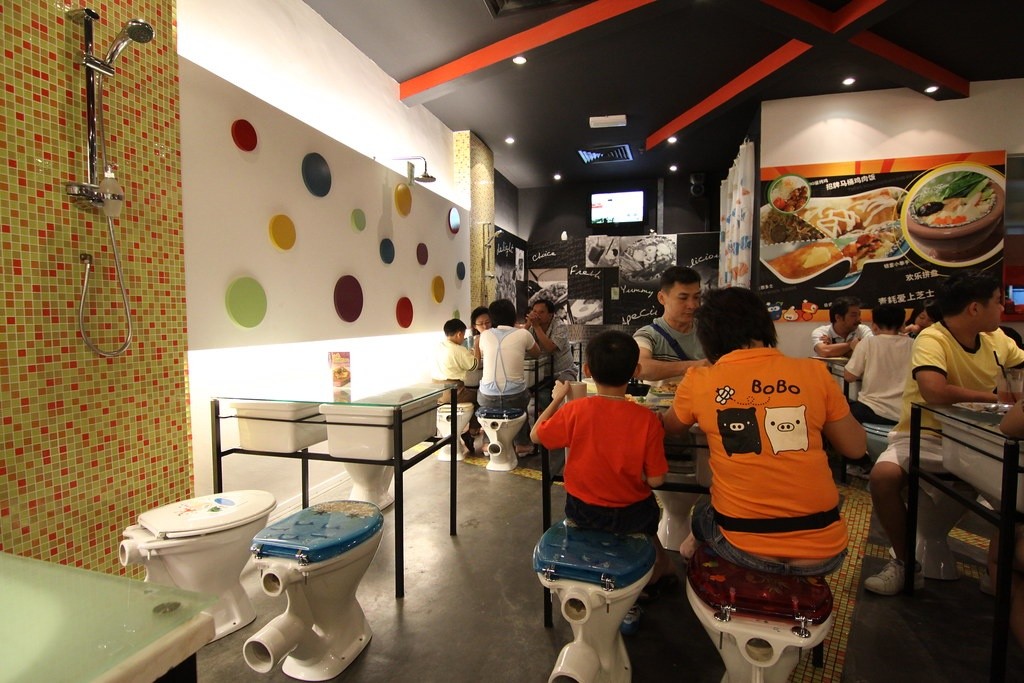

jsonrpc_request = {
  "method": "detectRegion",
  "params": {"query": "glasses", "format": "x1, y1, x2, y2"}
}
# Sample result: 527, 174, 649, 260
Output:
475, 320, 491, 326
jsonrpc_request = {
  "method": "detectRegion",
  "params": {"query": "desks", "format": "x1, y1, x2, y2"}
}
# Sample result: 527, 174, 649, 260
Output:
474, 339, 854, 627
211, 384, 460, 599
904, 400, 1024, 650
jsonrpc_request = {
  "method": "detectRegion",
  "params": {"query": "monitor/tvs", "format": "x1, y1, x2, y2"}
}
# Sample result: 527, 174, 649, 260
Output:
590, 191, 645, 223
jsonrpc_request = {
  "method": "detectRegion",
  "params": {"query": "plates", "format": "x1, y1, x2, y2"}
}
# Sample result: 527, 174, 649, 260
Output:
648, 397, 674, 409
649, 391, 676, 396
953, 402, 1014, 416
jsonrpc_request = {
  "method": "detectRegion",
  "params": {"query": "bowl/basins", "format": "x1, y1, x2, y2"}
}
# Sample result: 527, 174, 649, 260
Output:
906, 182, 1005, 263
625, 383, 651, 397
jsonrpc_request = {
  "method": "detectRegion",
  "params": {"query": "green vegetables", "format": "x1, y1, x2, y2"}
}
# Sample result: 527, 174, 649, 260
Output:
910, 170, 990, 210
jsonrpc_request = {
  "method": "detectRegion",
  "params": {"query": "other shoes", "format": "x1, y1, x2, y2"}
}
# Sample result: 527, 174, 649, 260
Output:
622, 592, 653, 624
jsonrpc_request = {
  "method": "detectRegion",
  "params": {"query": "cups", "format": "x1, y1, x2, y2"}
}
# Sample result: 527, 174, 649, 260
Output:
564, 381, 587, 405
997, 367, 1024, 404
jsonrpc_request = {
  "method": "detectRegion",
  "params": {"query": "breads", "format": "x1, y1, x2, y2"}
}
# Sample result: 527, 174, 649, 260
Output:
769, 191, 918, 280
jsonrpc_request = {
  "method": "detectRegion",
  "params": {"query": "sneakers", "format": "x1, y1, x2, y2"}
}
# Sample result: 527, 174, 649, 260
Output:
980, 568, 993, 594
863, 557, 924, 595
460, 430, 475, 455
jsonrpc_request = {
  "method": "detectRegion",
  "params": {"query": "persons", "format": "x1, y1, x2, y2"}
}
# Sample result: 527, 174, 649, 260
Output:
529, 266, 869, 633
811, 267, 1024, 656
434, 299, 578, 457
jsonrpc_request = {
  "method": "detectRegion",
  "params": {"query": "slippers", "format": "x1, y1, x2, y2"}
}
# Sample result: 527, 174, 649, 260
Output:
517, 446, 540, 457
484, 450, 490, 456
